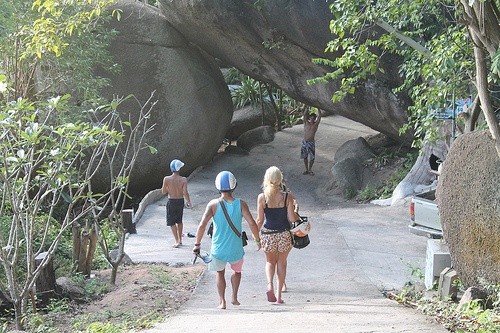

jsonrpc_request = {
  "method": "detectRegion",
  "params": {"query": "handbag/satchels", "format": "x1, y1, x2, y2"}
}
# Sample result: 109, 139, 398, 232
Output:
290, 212, 310, 249
242, 231, 248, 247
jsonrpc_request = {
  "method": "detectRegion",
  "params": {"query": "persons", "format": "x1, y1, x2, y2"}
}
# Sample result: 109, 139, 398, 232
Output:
161, 159, 192, 248
300, 107, 322, 176
252, 165, 299, 304
193, 171, 261, 309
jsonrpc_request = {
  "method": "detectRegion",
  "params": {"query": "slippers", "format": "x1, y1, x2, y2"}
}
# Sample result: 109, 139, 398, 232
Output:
267, 291, 277, 302
277, 300, 284, 303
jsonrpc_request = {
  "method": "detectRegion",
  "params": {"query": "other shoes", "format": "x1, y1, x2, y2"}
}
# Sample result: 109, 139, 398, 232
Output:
303, 170, 315, 176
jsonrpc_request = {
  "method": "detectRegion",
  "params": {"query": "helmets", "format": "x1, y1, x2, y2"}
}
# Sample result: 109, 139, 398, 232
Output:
215, 171, 236, 190
290, 220, 311, 237
170, 159, 184, 172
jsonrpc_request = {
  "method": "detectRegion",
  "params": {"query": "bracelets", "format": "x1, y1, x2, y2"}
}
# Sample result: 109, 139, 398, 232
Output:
256, 237, 260, 242
195, 244, 200, 246
